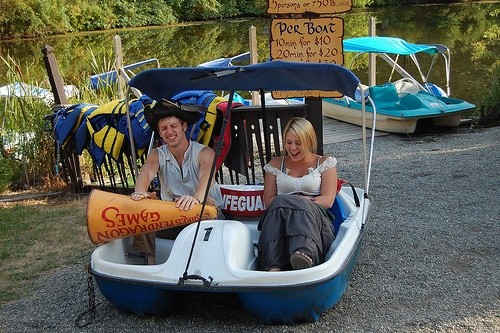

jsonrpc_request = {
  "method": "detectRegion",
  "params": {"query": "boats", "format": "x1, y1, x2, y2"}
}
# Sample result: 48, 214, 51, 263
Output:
0, 81, 80, 164
285, 36, 476, 135
87, 59, 376, 324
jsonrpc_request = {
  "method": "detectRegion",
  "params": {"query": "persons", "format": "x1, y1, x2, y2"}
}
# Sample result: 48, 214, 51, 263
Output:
259, 117, 336, 271
130, 105, 225, 265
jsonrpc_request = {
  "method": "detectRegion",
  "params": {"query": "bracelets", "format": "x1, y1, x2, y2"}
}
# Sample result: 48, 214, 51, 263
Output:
192, 197, 200, 203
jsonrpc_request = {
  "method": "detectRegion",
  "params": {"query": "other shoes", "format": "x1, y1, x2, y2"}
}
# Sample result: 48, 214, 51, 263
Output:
290, 250, 315, 270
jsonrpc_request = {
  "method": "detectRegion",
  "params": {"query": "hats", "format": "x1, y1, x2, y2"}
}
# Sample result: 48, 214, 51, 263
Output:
144, 100, 203, 139
